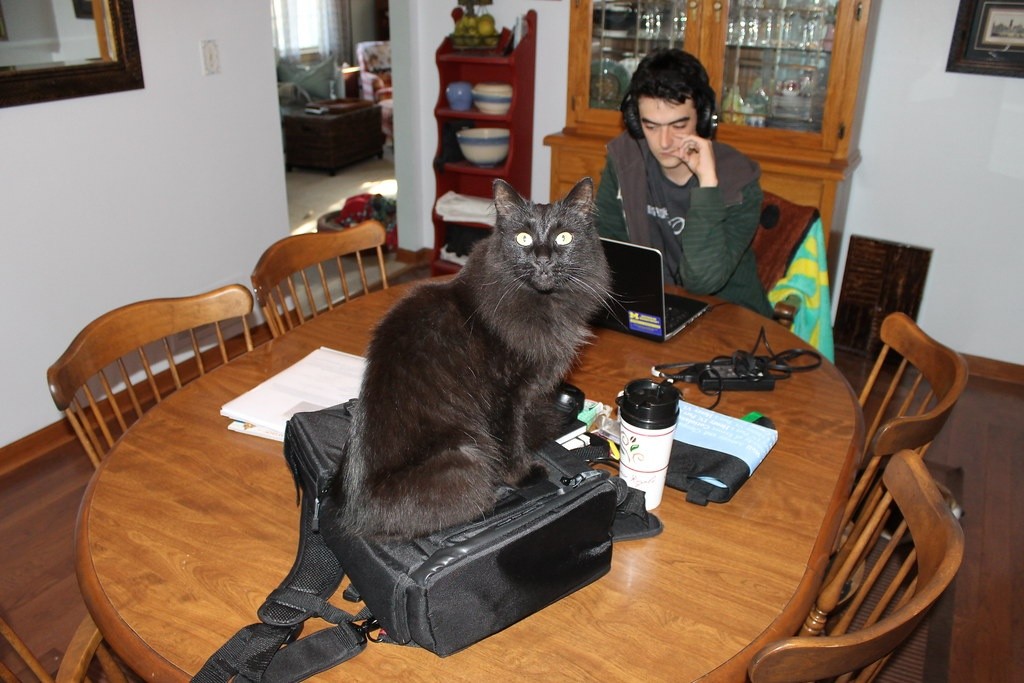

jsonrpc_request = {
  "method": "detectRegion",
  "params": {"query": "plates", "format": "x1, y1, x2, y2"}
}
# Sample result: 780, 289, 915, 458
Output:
590, 59, 629, 110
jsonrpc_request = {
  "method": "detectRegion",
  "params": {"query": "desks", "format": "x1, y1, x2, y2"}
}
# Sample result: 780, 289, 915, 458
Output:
75, 274, 862, 683
282, 98, 386, 178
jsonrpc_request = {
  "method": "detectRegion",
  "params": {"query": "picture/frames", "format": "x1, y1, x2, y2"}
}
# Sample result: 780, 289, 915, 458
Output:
945, 1, 1022, 80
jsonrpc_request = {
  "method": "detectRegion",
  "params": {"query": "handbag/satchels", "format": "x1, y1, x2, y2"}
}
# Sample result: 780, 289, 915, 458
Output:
280, 396, 613, 658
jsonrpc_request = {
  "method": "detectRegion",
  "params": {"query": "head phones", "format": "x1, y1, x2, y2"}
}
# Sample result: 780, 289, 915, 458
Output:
622, 85, 719, 141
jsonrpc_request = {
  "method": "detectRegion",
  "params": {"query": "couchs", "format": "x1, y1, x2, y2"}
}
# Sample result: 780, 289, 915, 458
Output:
358, 38, 393, 102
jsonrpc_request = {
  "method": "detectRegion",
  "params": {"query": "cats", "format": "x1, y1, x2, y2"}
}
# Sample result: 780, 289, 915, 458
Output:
327, 178, 632, 539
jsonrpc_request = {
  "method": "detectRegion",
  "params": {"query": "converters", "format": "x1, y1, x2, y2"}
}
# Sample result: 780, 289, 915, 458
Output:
697, 361, 774, 392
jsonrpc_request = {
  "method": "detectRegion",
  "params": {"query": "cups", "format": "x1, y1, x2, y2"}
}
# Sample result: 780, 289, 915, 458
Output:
551, 381, 585, 419
615, 379, 680, 511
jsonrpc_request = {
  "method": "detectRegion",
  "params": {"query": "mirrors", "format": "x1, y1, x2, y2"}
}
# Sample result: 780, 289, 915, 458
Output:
0, 0, 144, 107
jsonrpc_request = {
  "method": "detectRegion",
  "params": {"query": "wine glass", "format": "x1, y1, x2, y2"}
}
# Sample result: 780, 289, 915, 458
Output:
638, 0, 687, 41
726, 0, 827, 48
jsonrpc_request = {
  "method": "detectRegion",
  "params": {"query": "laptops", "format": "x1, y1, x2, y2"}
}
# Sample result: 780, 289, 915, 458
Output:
587, 236, 710, 342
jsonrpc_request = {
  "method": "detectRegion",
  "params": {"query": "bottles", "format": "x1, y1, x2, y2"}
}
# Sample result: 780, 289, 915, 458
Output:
445, 82, 472, 111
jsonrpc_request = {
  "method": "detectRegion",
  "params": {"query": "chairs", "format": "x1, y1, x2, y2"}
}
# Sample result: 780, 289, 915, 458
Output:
830, 311, 968, 552
249, 218, 389, 338
740, 190, 821, 325
744, 449, 964, 683
1, 615, 131, 683
46, 283, 256, 473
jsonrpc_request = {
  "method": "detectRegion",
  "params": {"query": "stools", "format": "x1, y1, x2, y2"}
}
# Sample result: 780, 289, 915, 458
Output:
378, 99, 393, 150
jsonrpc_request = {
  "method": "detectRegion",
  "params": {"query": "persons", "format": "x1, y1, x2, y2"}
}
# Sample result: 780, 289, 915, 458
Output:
593, 48, 778, 319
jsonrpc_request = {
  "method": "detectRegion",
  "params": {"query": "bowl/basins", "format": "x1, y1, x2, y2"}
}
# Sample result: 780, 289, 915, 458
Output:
457, 128, 509, 168
472, 83, 512, 115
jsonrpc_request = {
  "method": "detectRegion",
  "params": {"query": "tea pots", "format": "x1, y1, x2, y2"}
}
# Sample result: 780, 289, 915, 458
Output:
592, 0, 637, 38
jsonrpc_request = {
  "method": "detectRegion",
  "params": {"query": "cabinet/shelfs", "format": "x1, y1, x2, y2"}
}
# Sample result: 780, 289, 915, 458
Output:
432, 11, 539, 273
544, 1, 872, 254
